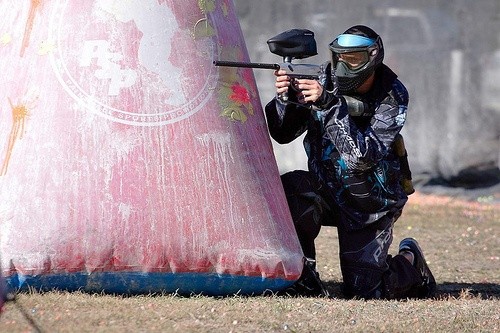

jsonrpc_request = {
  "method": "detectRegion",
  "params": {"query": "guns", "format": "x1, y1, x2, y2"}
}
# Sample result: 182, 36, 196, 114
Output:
212, 28, 380, 116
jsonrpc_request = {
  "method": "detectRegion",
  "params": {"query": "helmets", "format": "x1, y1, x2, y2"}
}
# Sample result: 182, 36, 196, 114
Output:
329, 25, 384, 91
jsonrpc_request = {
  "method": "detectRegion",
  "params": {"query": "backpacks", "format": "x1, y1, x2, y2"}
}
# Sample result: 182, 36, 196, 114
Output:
396, 133, 415, 195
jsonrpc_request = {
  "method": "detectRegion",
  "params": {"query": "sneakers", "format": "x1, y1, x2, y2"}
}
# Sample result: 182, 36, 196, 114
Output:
399, 238, 437, 288
287, 272, 319, 296
